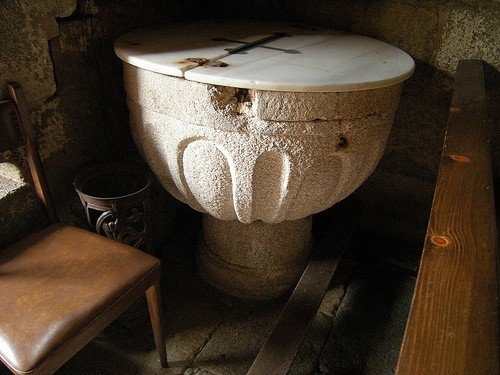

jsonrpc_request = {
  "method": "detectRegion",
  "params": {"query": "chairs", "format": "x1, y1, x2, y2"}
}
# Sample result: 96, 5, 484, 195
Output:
0, 80, 169, 375
